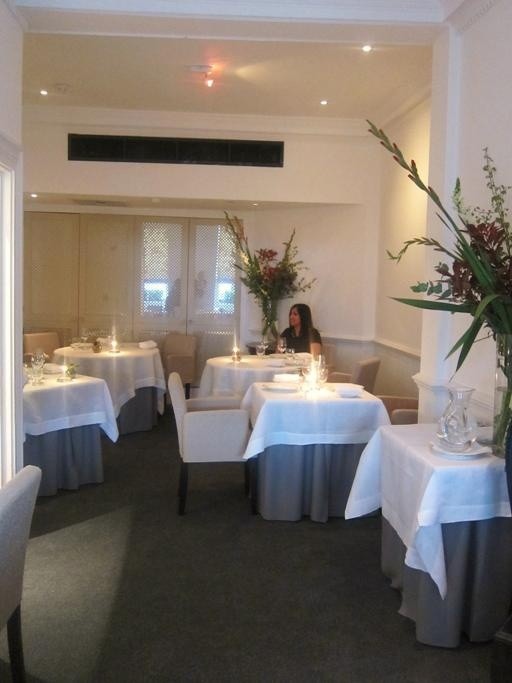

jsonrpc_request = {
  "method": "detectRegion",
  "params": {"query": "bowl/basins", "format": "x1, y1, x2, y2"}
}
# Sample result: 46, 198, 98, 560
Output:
335, 383, 365, 397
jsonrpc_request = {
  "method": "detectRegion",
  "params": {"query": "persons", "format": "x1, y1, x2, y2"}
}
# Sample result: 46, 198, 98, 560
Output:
277, 303, 322, 361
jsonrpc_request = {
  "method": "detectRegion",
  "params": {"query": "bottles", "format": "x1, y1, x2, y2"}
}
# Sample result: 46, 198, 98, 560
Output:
93, 341, 102, 352
439, 384, 477, 450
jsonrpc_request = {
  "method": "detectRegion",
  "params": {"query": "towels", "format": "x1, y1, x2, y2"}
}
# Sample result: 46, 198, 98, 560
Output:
138, 338, 158, 349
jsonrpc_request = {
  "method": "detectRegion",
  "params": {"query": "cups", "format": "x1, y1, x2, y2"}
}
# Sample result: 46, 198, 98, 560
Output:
231, 350, 241, 363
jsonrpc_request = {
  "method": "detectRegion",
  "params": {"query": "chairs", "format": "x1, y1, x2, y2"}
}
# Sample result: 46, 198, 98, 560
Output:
327, 356, 381, 393
0, 458, 45, 682
374, 388, 420, 423
24, 330, 62, 363
160, 331, 199, 396
164, 367, 257, 514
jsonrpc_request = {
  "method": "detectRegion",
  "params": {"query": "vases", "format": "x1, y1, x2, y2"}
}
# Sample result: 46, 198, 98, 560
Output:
259, 296, 281, 345
490, 329, 512, 460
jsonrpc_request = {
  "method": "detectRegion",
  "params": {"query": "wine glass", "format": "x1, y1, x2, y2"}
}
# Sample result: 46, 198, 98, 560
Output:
255, 335, 299, 368
300, 352, 329, 391
27, 350, 45, 386
69, 325, 90, 352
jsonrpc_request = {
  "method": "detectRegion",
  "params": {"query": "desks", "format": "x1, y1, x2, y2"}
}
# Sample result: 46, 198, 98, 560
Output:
245, 340, 276, 353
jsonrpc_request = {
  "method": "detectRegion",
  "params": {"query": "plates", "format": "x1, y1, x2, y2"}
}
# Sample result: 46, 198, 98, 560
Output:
44, 366, 66, 373
260, 381, 296, 392
428, 442, 494, 459
71, 342, 92, 348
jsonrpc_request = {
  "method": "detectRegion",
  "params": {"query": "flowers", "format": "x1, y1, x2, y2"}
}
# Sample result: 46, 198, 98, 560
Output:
360, 107, 512, 386
221, 210, 314, 297
